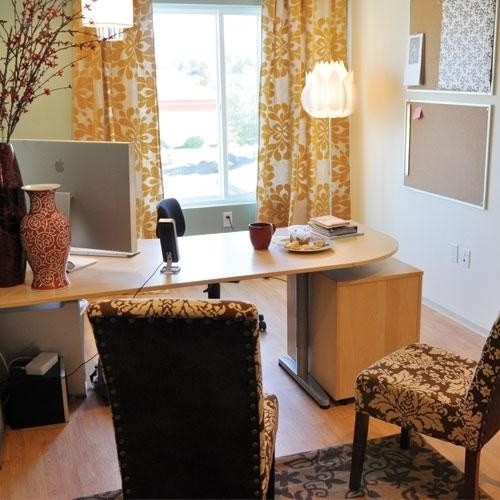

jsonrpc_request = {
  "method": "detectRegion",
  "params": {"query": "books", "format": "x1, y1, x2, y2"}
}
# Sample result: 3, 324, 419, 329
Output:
307, 214, 364, 240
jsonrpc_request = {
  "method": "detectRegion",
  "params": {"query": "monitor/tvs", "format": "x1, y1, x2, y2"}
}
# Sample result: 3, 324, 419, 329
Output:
0, 138, 139, 275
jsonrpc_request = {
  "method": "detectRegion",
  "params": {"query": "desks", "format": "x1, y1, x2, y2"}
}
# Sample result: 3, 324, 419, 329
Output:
0, 220, 398, 409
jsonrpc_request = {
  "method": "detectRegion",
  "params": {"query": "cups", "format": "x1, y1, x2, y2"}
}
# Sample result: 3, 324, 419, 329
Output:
248, 223, 274, 252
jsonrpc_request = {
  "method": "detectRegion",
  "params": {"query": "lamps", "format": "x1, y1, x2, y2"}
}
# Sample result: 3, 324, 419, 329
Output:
81, 0, 133, 41
301, 61, 357, 216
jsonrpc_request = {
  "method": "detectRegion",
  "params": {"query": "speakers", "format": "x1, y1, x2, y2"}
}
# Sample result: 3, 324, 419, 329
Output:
2, 354, 70, 429
158, 218, 181, 274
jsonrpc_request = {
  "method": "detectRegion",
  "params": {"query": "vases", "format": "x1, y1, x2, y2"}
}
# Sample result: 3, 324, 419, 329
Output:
0, 144, 26, 289
20, 183, 72, 291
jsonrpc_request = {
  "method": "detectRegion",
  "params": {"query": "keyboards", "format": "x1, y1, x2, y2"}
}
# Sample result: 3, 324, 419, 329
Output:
70, 247, 143, 258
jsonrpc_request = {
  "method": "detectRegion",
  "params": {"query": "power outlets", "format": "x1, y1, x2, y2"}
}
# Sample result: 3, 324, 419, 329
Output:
222, 212, 233, 229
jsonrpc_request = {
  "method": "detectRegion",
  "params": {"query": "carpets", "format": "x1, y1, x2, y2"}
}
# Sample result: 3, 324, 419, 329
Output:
73, 433, 493, 500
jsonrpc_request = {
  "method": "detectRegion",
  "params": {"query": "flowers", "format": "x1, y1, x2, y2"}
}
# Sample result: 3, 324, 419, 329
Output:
0, 12, 136, 146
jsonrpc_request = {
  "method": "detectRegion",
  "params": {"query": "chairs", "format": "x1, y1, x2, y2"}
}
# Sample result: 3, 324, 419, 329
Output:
349, 313, 500, 500
88, 298, 278, 500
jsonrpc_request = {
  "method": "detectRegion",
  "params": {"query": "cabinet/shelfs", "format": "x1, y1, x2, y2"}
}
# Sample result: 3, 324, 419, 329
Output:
287, 256, 424, 401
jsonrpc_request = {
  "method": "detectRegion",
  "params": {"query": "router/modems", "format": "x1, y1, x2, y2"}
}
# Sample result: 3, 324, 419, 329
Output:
25, 350, 59, 376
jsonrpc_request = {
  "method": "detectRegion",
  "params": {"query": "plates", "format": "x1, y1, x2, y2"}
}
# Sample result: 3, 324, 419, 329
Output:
279, 239, 330, 254
